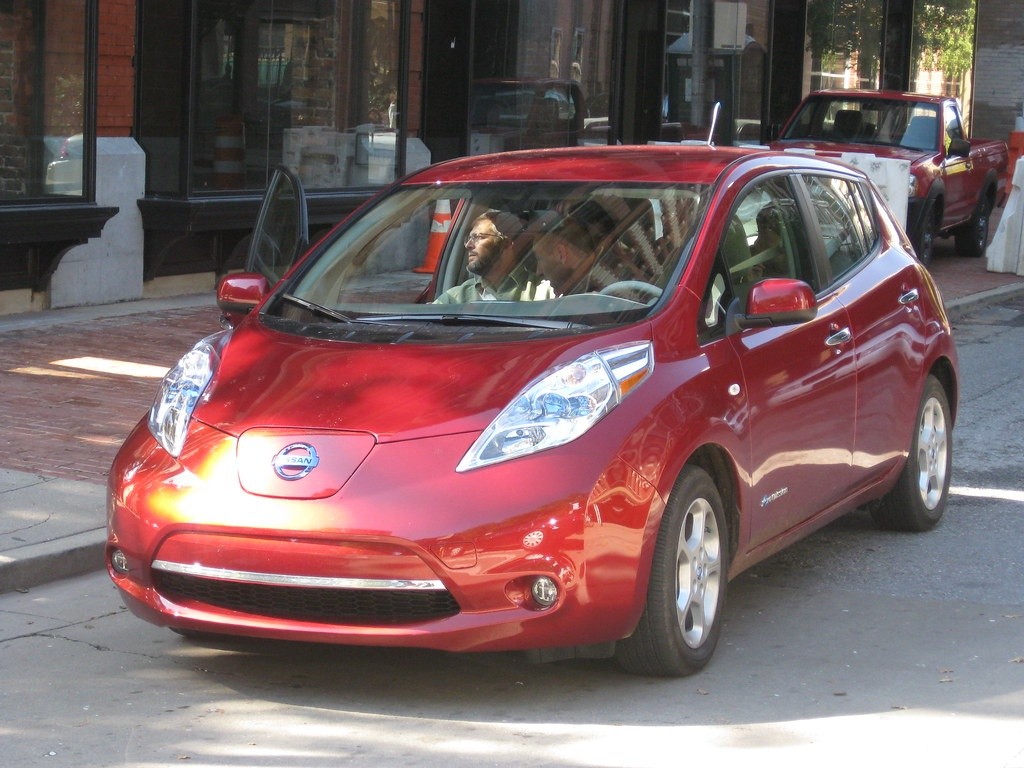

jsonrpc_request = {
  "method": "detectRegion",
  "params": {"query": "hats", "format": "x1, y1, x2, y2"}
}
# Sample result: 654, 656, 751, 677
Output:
758, 201, 783, 236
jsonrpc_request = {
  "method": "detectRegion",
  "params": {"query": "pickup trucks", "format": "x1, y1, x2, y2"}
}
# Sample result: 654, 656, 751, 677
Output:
766, 89, 1009, 272
446, 76, 586, 166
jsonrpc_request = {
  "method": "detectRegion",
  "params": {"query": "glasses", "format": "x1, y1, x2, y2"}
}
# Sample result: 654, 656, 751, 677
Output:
463, 233, 503, 244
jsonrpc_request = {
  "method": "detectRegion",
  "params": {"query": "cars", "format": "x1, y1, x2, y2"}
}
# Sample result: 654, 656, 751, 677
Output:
103, 144, 960, 681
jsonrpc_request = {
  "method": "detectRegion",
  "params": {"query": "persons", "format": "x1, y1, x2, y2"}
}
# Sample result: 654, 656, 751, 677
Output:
530, 215, 658, 303
424, 208, 558, 304
749, 201, 794, 281
